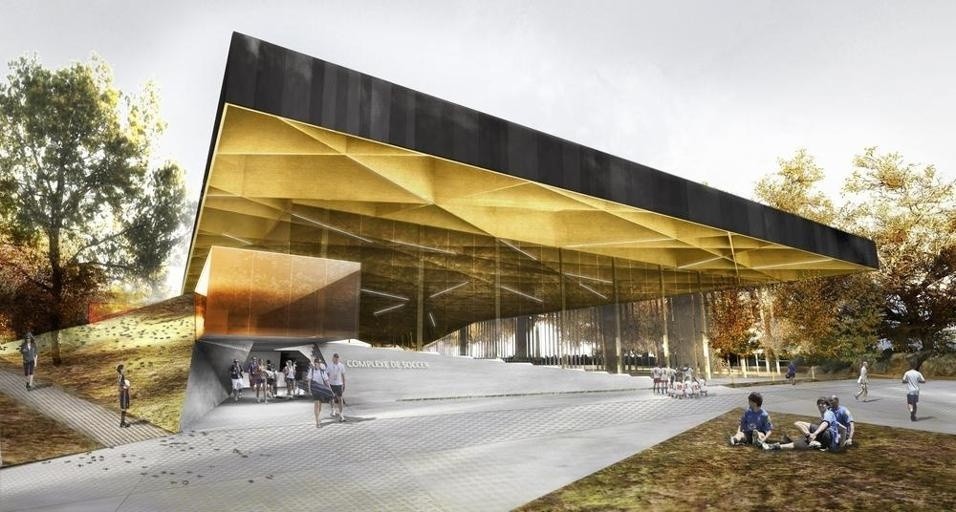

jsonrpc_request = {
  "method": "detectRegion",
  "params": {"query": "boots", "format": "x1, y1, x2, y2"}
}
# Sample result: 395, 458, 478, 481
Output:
806, 434, 821, 447
762, 441, 782, 451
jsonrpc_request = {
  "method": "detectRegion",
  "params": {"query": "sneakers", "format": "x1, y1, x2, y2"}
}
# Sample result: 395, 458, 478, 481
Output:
911, 411, 917, 421
330, 410, 345, 423
752, 430, 762, 447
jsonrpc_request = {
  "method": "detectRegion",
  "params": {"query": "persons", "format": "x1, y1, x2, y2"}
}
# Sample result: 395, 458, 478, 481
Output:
757, 396, 847, 451
828, 394, 855, 448
853, 361, 870, 402
116, 364, 132, 428
22, 331, 39, 391
648, 362, 708, 399
785, 361, 799, 385
901, 359, 927, 419
728, 391, 772, 446
227, 356, 303, 402
306, 352, 347, 430
